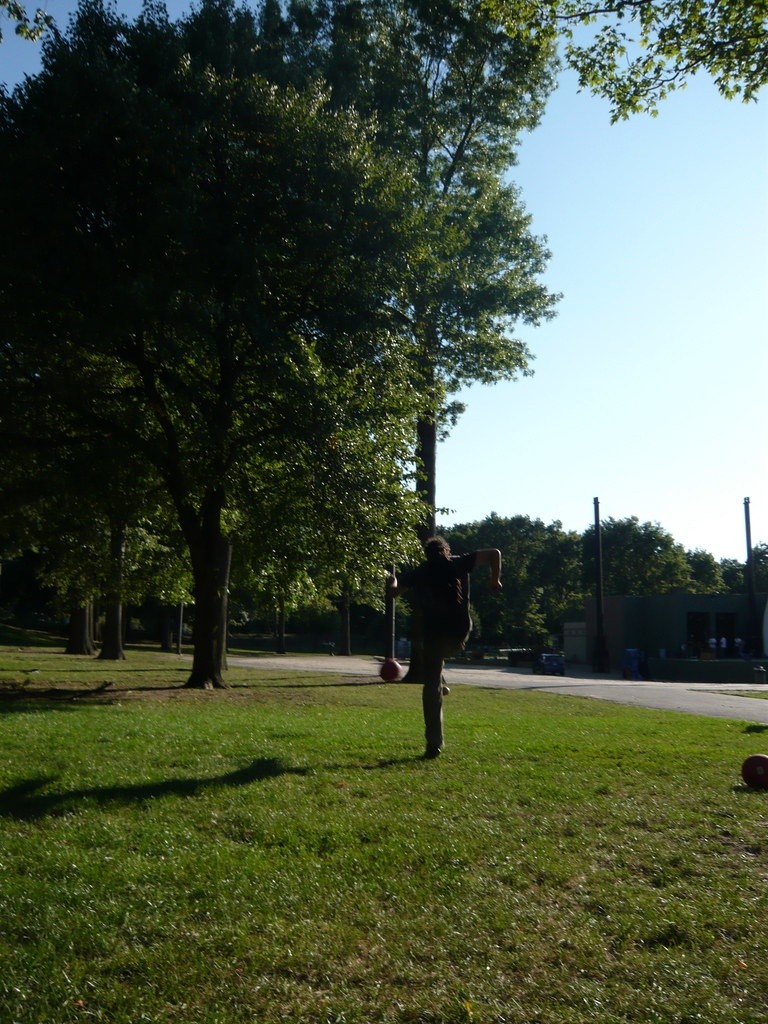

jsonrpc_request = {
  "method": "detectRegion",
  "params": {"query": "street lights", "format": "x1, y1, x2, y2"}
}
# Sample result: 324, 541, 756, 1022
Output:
593, 496, 611, 674
742, 496, 764, 658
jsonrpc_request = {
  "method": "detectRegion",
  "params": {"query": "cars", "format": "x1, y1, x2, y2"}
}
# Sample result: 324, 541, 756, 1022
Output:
532, 651, 566, 676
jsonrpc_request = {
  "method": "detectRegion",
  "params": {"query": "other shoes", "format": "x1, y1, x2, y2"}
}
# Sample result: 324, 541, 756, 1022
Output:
424, 742, 442, 759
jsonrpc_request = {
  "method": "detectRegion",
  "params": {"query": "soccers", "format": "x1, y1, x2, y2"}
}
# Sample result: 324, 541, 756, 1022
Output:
741, 753, 768, 791
377, 659, 402, 683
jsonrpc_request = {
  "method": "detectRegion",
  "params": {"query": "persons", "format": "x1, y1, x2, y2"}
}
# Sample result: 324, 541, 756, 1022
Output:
720, 636, 728, 657
734, 636, 743, 657
708, 636, 717, 649
383, 536, 504, 761
